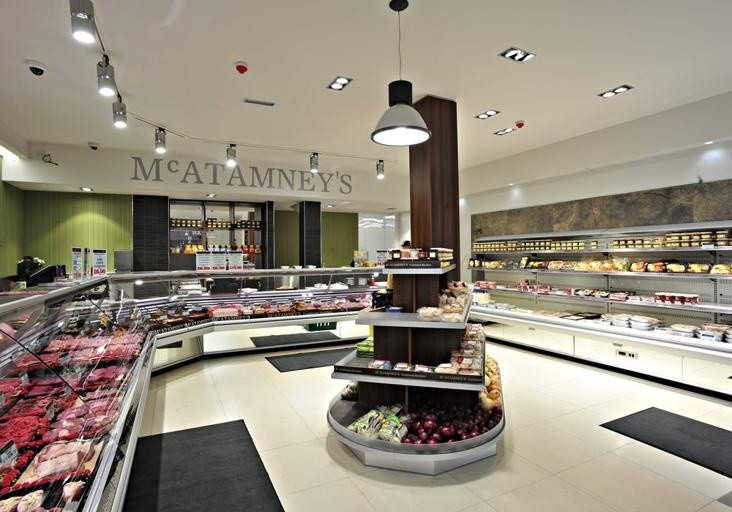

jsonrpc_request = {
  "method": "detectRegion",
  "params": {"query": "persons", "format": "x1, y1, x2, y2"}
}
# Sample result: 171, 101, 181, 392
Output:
401, 240, 411, 249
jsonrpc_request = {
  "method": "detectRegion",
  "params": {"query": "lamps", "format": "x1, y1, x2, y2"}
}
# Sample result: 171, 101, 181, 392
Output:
370, 0, 433, 148
67, 0, 401, 182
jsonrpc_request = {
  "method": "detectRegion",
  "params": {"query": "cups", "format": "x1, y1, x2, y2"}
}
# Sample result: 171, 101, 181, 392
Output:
654, 291, 700, 305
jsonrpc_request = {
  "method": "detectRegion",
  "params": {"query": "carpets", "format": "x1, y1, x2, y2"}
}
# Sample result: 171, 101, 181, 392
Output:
248, 331, 339, 349
265, 346, 361, 373
120, 419, 285, 510
598, 406, 731, 481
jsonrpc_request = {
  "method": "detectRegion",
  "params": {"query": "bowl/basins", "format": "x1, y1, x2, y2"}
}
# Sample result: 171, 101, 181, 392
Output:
308, 265, 316, 269
281, 266, 289, 270
294, 266, 302, 270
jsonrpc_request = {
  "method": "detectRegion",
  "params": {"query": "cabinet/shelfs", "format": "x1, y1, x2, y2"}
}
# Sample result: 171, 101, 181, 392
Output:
327, 261, 508, 478
168, 198, 263, 294
109, 255, 391, 373
0, 260, 158, 511
464, 227, 732, 396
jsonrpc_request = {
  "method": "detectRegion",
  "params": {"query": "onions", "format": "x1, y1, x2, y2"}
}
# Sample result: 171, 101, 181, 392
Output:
402, 405, 502, 444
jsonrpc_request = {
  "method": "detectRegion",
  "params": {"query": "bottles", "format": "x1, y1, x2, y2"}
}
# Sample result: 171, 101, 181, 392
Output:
171, 242, 261, 253
225, 257, 230, 270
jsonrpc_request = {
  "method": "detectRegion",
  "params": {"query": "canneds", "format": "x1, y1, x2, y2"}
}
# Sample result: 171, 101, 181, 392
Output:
170, 218, 202, 227
472, 239, 598, 253
207, 217, 232, 227
236, 219, 261, 229
655, 291, 699, 305
376, 247, 454, 269
612, 230, 732, 249
